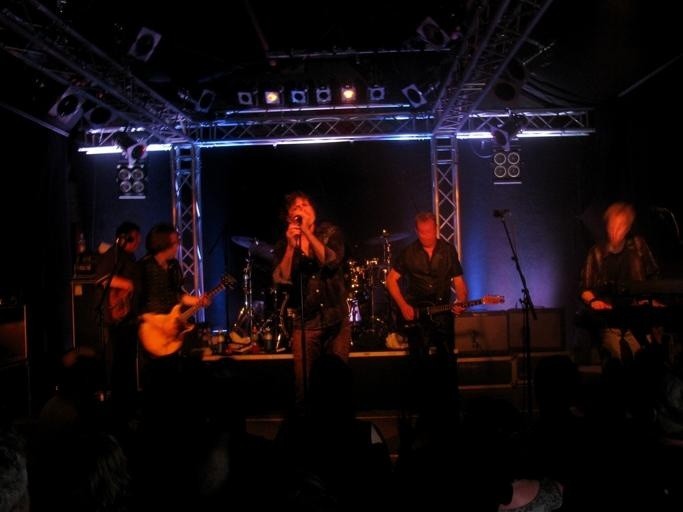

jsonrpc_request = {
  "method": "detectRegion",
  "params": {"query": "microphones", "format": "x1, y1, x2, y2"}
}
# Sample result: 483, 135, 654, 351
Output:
292, 215, 303, 249
492, 208, 512, 218
120, 232, 134, 243
649, 206, 671, 214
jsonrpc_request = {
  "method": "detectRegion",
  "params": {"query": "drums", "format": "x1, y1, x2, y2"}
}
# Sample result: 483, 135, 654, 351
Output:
264, 284, 295, 350
345, 258, 385, 288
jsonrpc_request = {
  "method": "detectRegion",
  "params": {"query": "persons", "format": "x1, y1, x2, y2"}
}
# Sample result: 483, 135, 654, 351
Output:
0, 323, 682, 511
389, 211, 468, 359
128, 222, 213, 338
578, 202, 661, 371
270, 190, 351, 399
98, 219, 143, 359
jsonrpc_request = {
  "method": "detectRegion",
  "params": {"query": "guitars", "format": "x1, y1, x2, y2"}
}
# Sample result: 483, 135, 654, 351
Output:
138, 274, 235, 356
392, 294, 504, 335
101, 287, 133, 326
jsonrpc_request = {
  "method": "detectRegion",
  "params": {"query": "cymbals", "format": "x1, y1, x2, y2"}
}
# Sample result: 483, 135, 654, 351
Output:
233, 236, 276, 266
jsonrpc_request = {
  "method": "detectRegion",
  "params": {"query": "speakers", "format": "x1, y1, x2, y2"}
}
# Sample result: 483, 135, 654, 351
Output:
507, 309, 566, 353
453, 311, 509, 356
0, 303, 29, 369
